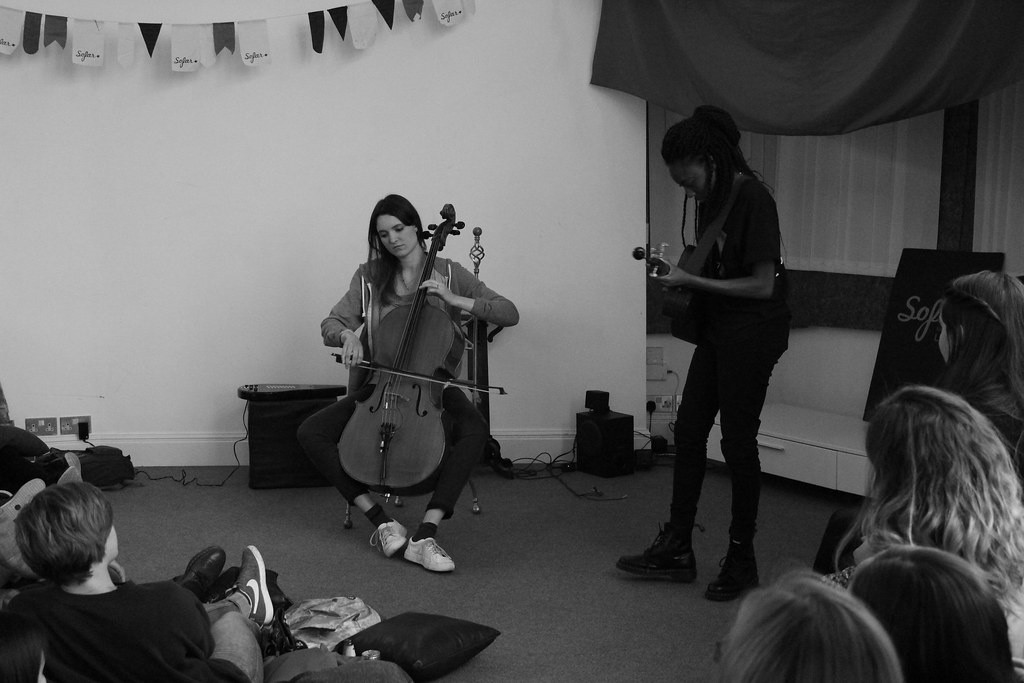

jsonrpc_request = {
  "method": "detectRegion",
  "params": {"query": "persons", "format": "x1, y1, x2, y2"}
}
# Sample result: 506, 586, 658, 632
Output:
715, 270, 1023, 683
616, 108, 791, 603
0, 384, 274, 683
298, 193, 520, 572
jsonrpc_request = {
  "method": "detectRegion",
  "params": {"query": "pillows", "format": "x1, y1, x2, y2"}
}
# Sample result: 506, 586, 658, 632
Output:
337, 610, 500, 683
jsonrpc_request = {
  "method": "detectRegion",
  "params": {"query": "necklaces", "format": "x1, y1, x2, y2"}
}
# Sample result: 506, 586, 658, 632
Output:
400, 272, 409, 290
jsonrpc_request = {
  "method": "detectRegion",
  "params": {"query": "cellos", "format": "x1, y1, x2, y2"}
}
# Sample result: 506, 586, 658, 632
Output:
339, 204, 465, 552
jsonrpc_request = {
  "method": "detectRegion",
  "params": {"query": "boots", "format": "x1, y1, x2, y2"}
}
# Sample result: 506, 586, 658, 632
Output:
616, 522, 698, 584
704, 535, 758, 602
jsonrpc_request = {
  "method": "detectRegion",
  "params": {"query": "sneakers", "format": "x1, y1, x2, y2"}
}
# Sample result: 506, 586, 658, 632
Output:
369, 517, 406, 557
404, 536, 455, 572
225, 543, 273, 625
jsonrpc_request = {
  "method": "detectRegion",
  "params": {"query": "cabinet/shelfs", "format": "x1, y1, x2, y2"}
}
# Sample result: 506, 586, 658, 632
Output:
707, 424, 879, 501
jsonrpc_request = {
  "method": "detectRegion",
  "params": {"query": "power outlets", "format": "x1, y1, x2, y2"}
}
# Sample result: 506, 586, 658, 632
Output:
60, 416, 92, 434
646, 395, 673, 413
25, 418, 57, 437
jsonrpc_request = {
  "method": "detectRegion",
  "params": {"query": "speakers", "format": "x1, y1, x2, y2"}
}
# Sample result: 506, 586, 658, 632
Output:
575, 390, 635, 476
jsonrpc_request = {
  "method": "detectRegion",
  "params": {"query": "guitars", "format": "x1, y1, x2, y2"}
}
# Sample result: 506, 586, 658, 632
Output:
641, 246, 721, 346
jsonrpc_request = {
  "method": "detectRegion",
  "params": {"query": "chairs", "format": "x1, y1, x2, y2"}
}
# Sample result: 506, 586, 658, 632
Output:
344, 322, 482, 530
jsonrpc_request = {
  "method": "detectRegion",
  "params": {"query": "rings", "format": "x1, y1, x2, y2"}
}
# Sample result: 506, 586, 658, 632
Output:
436, 285, 438, 288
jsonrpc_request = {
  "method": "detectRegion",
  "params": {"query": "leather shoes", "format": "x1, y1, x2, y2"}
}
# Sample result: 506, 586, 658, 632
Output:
173, 546, 226, 594
